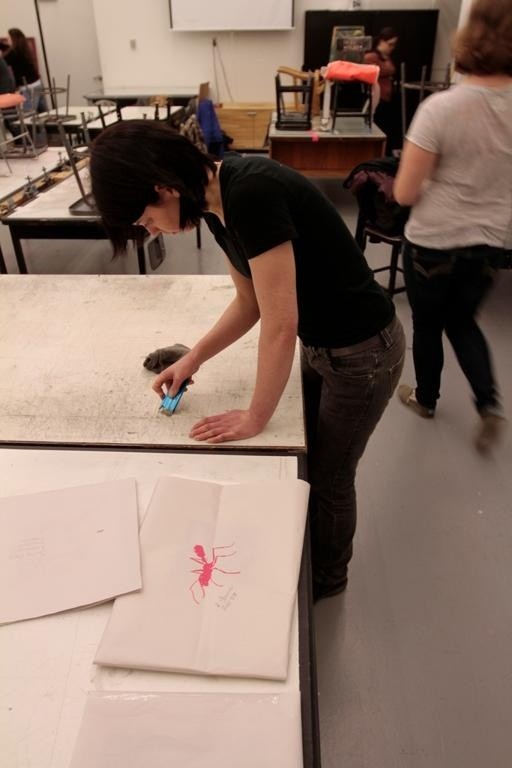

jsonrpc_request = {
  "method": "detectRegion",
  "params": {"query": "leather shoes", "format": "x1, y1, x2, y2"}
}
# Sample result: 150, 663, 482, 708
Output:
398, 382, 437, 420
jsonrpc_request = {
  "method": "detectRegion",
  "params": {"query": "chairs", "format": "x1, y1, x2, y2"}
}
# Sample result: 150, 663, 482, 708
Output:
266, 60, 452, 181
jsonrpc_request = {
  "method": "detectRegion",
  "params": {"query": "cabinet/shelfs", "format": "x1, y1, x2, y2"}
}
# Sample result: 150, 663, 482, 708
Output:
213, 102, 306, 151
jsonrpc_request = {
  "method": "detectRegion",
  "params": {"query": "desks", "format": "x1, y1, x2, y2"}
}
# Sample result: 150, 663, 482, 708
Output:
0, 443, 321, 768
1, 272, 307, 451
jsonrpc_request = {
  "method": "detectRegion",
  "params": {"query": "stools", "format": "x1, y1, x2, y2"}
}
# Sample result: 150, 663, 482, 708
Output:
357, 225, 407, 309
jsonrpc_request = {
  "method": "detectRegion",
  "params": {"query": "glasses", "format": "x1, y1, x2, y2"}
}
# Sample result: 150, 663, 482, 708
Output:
385, 42, 396, 47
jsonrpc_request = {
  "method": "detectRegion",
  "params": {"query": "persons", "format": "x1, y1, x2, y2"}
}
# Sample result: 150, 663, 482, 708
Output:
361, 26, 400, 127
394, 0, 510, 464
90, 119, 407, 606
0, 27, 42, 149
